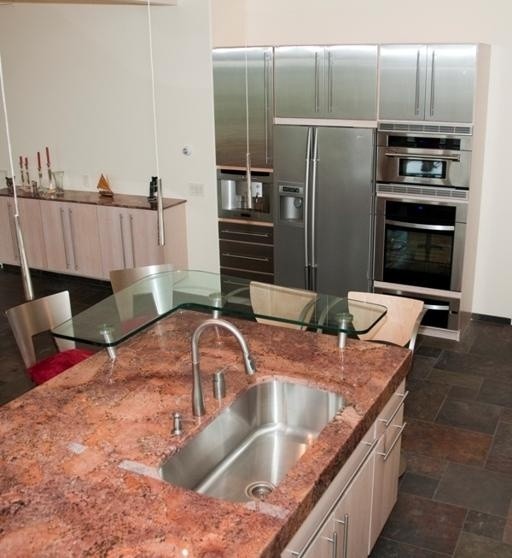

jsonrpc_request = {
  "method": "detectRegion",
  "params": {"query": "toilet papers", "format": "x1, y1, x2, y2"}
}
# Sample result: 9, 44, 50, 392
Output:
221, 179, 237, 211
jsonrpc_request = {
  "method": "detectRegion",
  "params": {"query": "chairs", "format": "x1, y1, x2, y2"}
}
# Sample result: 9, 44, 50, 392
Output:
4, 266, 174, 381
250, 280, 425, 368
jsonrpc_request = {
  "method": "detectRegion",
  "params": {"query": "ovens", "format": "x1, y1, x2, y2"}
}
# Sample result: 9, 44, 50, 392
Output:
216, 165, 271, 225
374, 121, 479, 331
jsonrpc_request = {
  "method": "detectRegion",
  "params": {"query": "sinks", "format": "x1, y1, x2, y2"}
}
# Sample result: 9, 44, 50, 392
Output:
155, 371, 353, 506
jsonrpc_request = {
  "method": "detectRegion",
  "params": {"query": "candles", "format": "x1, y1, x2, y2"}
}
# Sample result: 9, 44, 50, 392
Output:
17, 146, 52, 170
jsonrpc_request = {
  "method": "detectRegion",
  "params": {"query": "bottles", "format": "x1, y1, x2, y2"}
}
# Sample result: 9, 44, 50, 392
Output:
149, 176, 158, 200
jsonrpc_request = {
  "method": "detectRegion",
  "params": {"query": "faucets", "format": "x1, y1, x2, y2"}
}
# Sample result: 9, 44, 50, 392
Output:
190, 318, 257, 417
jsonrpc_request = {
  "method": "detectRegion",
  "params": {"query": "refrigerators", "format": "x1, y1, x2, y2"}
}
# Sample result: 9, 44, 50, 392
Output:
270, 123, 376, 300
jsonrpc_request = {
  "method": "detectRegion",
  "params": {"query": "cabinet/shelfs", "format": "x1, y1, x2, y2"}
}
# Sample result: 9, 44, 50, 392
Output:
273, 43, 477, 131
0, 196, 187, 315
275, 375, 408, 558
210, 45, 274, 174
219, 222, 273, 313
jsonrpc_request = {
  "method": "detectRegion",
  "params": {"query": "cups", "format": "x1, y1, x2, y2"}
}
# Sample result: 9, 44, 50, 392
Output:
52, 171, 64, 194
